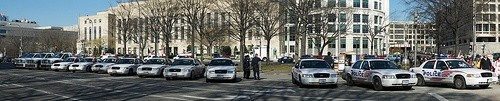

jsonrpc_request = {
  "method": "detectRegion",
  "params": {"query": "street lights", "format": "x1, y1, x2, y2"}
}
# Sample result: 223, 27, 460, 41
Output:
9, 23, 23, 58
482, 41, 486, 55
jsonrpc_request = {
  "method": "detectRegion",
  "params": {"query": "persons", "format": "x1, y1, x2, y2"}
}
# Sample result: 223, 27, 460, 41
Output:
394, 53, 435, 66
465, 54, 500, 84
251, 53, 262, 80
243, 55, 251, 79
324, 52, 334, 68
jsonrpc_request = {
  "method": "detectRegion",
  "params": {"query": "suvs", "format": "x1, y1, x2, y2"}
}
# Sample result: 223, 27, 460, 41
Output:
41, 52, 73, 70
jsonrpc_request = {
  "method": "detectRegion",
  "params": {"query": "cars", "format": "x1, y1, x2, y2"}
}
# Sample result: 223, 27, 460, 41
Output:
107, 58, 143, 76
342, 58, 418, 90
91, 57, 122, 73
291, 59, 338, 88
164, 58, 206, 81
410, 59, 495, 90
69, 56, 101, 72
144, 55, 159, 63
301, 55, 338, 63
278, 56, 295, 64
51, 56, 85, 72
204, 58, 238, 82
137, 58, 172, 78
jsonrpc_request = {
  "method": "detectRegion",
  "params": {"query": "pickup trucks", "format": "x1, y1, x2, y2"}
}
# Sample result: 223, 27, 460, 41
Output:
384, 54, 401, 62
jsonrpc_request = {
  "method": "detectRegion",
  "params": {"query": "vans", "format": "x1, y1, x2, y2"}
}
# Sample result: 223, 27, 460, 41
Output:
12, 53, 32, 68
24, 52, 40, 70
